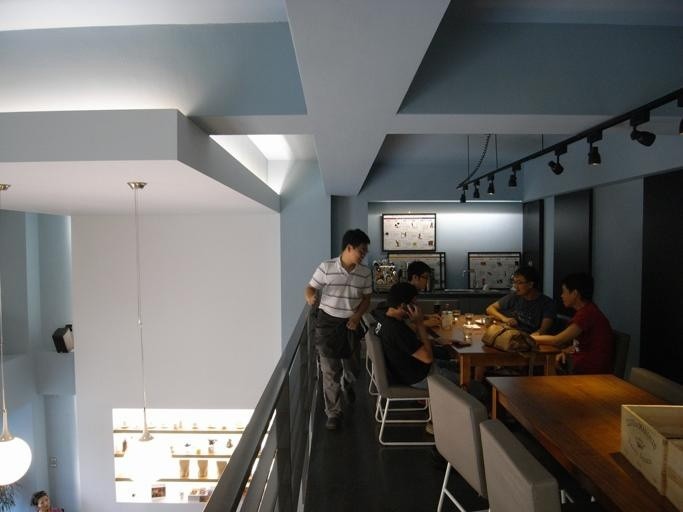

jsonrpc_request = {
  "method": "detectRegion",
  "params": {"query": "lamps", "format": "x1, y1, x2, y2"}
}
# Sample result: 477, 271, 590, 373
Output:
456, 87, 683, 204
0, 367, 32, 486
125, 181, 171, 479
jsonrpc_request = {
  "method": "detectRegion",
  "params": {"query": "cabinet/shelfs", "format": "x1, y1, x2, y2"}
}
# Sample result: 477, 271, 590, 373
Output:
114, 428, 277, 504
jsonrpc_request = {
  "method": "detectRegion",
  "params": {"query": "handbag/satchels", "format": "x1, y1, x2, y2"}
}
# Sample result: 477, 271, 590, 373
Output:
481, 324, 541, 353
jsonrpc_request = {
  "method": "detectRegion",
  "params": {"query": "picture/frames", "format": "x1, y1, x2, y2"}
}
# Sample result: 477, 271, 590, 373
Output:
468, 251, 521, 290
388, 252, 448, 290
383, 213, 436, 253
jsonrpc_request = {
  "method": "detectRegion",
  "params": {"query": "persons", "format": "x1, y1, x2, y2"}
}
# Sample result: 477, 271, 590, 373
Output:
29, 490, 66, 512
378, 281, 484, 435
484, 265, 557, 338
304, 227, 375, 431
372, 260, 451, 361
532, 275, 613, 378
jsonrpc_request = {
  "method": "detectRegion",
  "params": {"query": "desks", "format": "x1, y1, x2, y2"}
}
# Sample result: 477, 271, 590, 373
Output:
373, 290, 511, 299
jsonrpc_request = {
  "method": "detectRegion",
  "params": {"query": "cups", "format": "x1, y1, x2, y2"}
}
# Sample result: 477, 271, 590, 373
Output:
438, 308, 491, 343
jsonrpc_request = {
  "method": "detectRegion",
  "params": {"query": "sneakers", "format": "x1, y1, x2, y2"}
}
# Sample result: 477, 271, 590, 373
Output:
426, 423, 434, 433
325, 417, 340, 429
345, 388, 356, 402
